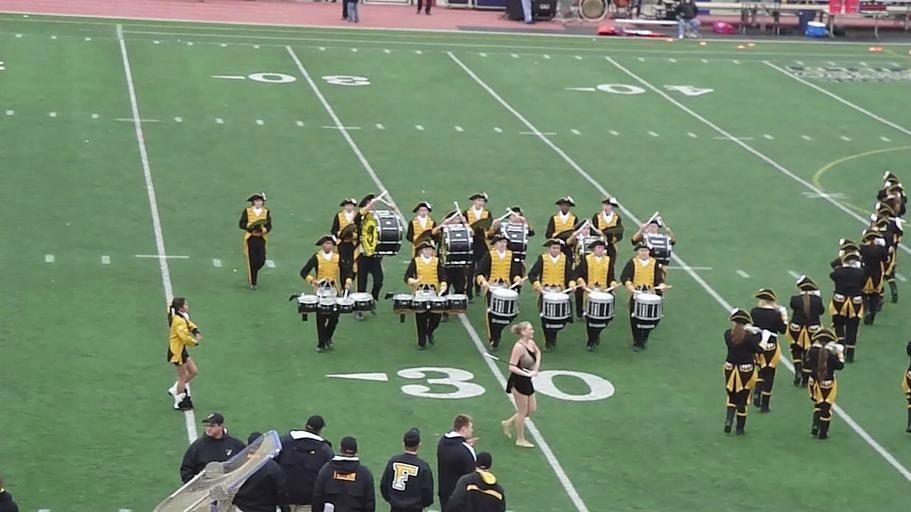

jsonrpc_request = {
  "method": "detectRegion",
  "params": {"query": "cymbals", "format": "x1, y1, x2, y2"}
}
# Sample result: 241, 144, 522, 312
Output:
248, 219, 267, 230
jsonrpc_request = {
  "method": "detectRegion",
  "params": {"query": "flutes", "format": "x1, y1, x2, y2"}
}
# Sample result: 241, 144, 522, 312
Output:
888, 217, 905, 224
743, 325, 779, 337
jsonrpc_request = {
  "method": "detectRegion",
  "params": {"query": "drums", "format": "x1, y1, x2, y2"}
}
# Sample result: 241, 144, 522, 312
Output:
296, 286, 374, 314
487, 288, 519, 317
362, 209, 404, 259
440, 223, 473, 270
501, 223, 529, 259
575, 236, 596, 262
634, 293, 664, 320
641, 233, 670, 264
393, 290, 467, 314
586, 292, 615, 321
543, 293, 573, 321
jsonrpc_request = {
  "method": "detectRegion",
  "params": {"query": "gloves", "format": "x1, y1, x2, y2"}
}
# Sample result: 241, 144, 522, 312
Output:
744, 326, 759, 334
759, 330, 770, 351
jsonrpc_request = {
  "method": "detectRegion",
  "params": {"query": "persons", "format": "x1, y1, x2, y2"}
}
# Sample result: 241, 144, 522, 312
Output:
676, 0, 701, 39
163, 296, 203, 411
437, 414, 506, 512
829, 170, 908, 362
181, 412, 376, 512
724, 275, 845, 438
301, 194, 396, 351
403, 192, 536, 348
342, 0, 349, 22
501, 320, 542, 447
900, 340, 911, 433
416, 0, 432, 16
528, 195, 675, 351
345, 0, 359, 23
1, 475, 20, 512
239, 193, 272, 291
381, 427, 434, 512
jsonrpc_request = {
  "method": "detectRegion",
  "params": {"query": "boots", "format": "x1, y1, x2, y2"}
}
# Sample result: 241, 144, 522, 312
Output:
839, 338, 855, 363
725, 380, 771, 435
864, 280, 898, 324
167, 381, 187, 410
794, 360, 811, 389
810, 409, 830, 439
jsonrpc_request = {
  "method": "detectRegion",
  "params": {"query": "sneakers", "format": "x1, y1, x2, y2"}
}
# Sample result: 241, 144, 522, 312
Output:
250, 285, 257, 289
355, 310, 376, 320
468, 292, 481, 303
440, 316, 447, 322
516, 440, 534, 447
317, 337, 334, 352
490, 337, 648, 351
502, 421, 511, 438
418, 332, 434, 349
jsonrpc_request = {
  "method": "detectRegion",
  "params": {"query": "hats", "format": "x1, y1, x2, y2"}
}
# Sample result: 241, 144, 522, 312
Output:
862, 173, 904, 242
248, 432, 261, 444
404, 428, 420, 448
476, 453, 491, 469
358, 195, 374, 207
201, 413, 223, 423
339, 199, 357, 207
839, 239, 862, 263
812, 329, 838, 344
245, 192, 267, 201
341, 437, 357, 454
728, 277, 817, 323
412, 193, 662, 251
306, 415, 325, 431
315, 236, 337, 245
